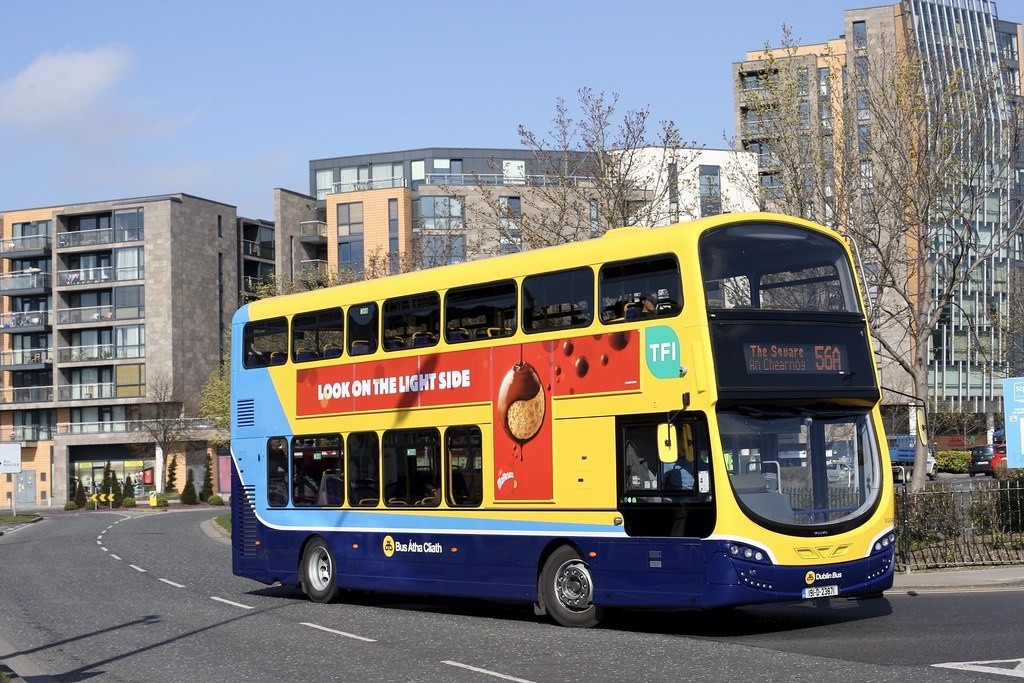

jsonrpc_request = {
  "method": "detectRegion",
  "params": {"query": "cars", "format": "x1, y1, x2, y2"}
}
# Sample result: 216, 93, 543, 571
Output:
826, 462, 854, 480
991, 444, 1007, 478
968, 444, 1004, 478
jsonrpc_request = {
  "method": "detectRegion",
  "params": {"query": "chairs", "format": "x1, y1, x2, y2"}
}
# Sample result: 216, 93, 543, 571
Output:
268, 301, 637, 365
293, 468, 473, 506
32, 352, 40, 363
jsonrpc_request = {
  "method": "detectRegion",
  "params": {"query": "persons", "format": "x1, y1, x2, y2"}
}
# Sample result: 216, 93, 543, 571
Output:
640, 288, 658, 314
662, 430, 706, 490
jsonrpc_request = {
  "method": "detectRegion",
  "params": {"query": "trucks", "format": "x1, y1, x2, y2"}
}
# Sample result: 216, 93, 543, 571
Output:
884, 433, 940, 483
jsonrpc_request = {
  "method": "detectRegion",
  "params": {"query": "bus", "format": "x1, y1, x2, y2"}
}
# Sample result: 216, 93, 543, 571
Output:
229, 212, 930, 628
